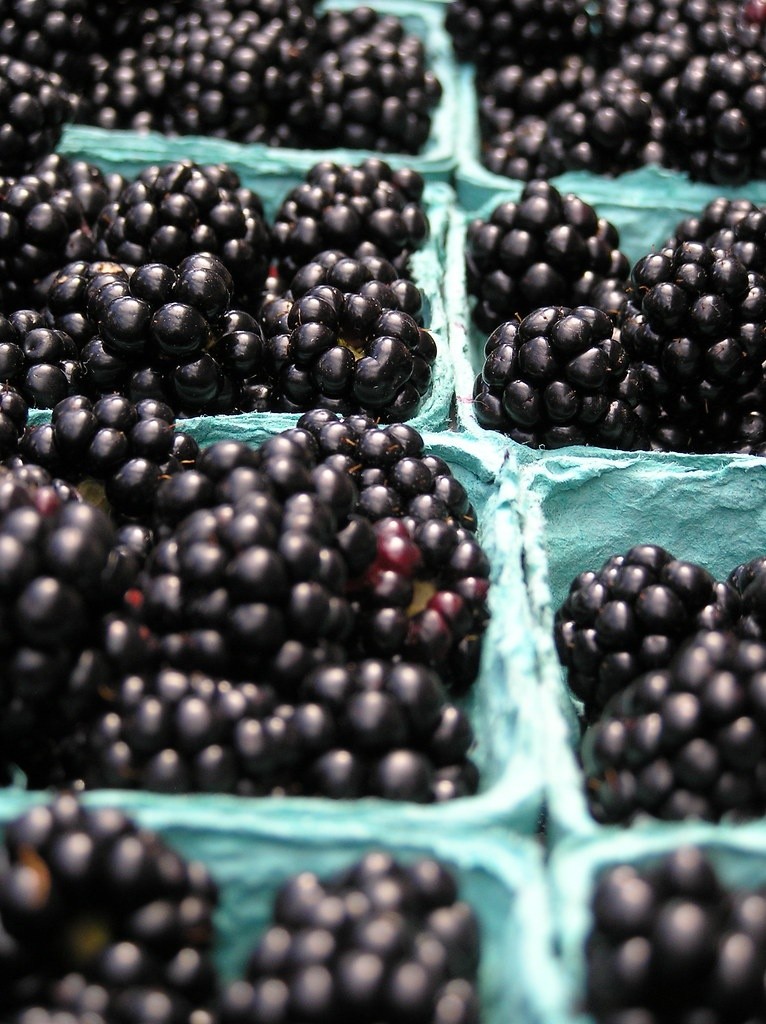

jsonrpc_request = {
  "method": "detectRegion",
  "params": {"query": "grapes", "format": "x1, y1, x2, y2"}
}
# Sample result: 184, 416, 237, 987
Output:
0, 0, 766, 1023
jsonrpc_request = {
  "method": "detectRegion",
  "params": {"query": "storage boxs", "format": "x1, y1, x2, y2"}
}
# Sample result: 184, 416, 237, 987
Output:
0, 0, 766, 1024
